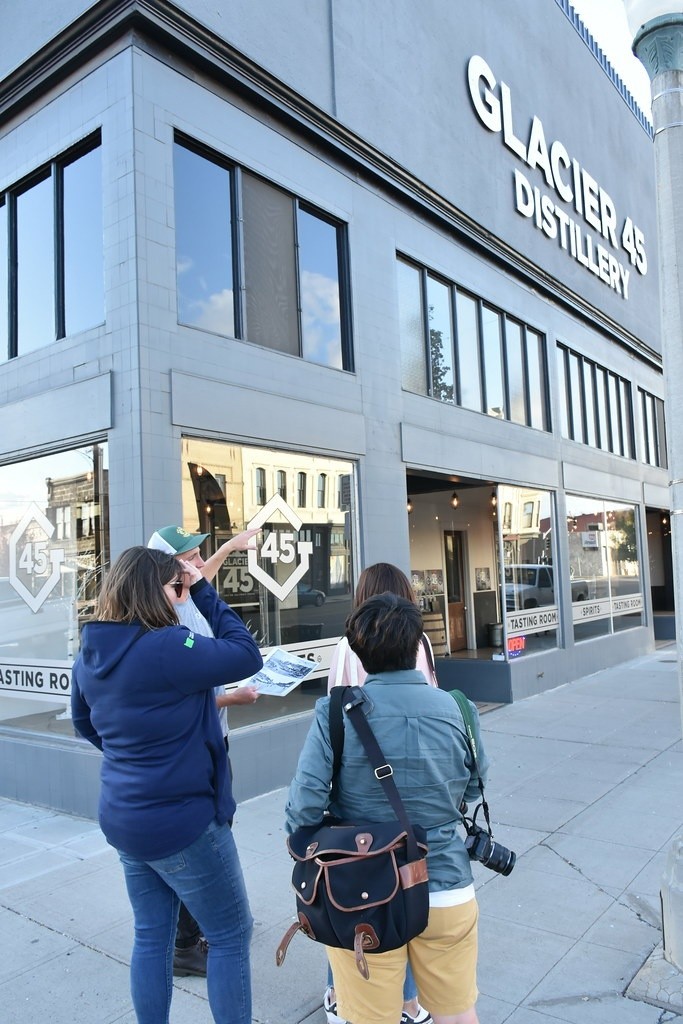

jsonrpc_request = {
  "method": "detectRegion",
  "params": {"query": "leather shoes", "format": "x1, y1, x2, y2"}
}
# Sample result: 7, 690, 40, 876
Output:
172, 938, 208, 978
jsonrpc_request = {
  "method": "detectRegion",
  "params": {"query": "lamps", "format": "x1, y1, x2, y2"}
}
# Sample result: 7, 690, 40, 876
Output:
491, 487, 497, 507
451, 490, 458, 510
407, 496, 412, 514
206, 499, 211, 512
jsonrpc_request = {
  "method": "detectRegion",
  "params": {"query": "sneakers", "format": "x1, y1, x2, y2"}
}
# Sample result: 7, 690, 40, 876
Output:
400, 1003, 434, 1024
323, 986, 347, 1024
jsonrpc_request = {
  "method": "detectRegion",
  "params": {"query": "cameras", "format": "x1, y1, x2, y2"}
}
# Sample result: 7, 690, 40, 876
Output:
464, 825, 516, 877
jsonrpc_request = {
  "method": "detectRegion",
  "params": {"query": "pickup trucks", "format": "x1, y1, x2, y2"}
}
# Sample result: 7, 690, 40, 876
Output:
498, 563, 590, 612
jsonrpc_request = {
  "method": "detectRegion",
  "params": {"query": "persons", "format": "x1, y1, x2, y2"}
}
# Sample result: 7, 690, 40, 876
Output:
146, 524, 264, 976
285, 595, 491, 1024
69, 543, 263, 1024
322, 562, 441, 1024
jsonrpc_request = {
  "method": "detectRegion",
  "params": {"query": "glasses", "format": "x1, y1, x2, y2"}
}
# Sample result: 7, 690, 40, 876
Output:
165, 581, 183, 598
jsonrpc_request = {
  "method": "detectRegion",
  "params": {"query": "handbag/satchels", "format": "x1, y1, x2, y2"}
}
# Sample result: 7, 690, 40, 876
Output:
275, 820, 429, 980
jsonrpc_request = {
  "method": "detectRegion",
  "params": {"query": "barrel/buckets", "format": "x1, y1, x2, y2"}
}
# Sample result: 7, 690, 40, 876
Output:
486, 622, 504, 647
422, 611, 447, 658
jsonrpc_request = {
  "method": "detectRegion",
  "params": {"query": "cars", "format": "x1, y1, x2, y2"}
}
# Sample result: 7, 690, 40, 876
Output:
252, 582, 327, 611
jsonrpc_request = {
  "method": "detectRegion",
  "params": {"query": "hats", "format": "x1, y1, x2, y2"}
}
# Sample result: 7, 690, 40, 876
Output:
147, 524, 211, 555
